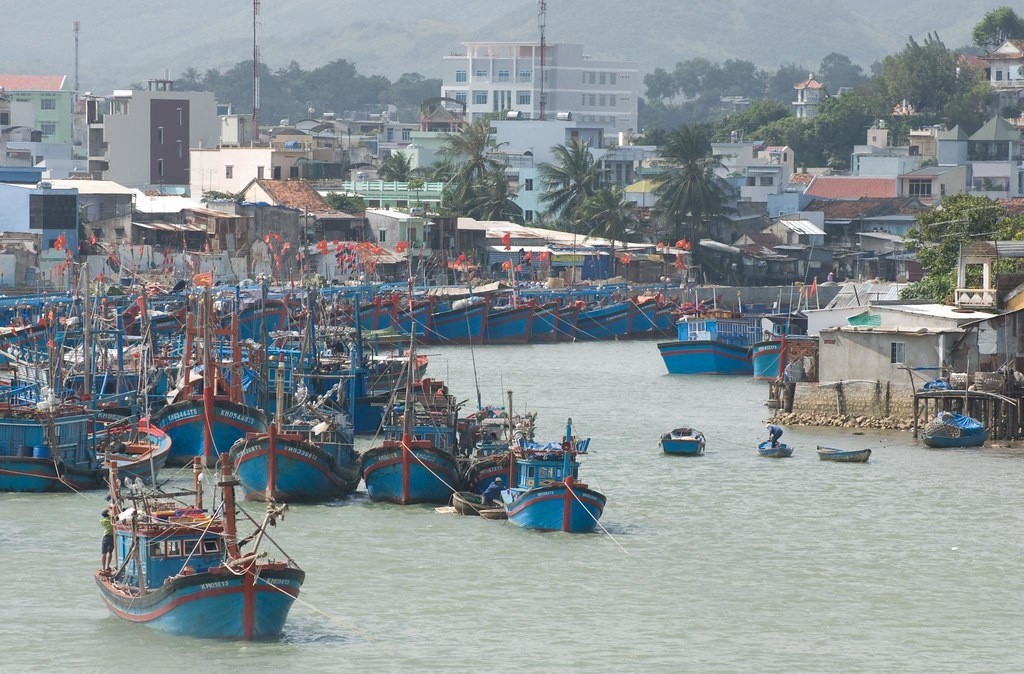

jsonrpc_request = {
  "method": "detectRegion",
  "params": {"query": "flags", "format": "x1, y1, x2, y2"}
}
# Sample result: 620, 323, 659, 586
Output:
796, 277, 816, 298
4, 232, 691, 352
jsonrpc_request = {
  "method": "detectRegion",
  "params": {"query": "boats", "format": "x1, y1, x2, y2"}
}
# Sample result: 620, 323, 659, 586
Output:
656, 283, 754, 374
659, 426, 705, 454
921, 411, 987, 448
228, 290, 363, 503
748, 236, 833, 379
93, 435, 305, 642
152, 285, 267, 466
0, 274, 173, 493
816, 446, 871, 463
0, 207, 722, 433
357, 321, 461, 506
757, 439, 792, 458
438, 327, 608, 532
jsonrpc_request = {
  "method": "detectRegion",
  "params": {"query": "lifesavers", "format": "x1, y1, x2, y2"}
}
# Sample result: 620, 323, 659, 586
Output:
562, 442, 571, 451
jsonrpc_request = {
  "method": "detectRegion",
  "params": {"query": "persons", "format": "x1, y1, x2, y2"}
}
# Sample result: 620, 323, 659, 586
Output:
100, 509, 118, 573
766, 424, 783, 448
826, 272, 834, 282
